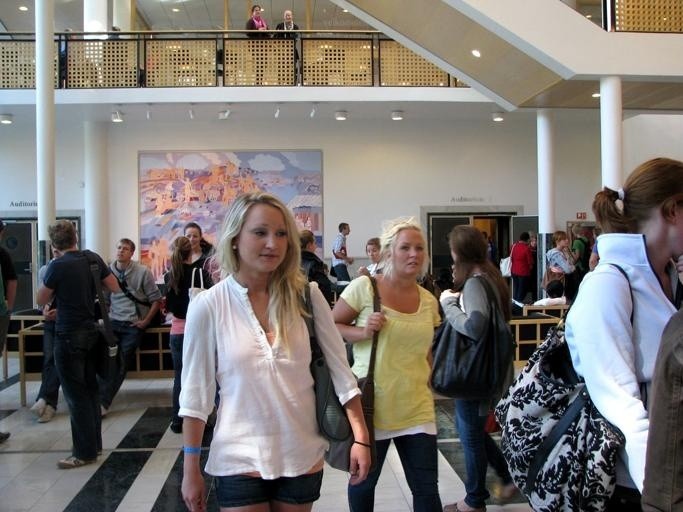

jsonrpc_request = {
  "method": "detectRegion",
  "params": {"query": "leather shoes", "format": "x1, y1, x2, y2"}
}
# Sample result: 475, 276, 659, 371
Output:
492, 483, 517, 505
442, 500, 488, 512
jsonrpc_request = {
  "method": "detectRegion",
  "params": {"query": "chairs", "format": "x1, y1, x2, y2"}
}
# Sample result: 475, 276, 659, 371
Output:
3, 50, 385, 90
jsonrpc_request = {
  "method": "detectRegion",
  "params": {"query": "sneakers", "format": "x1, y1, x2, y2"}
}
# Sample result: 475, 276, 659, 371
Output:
483, 410, 498, 433
57, 454, 98, 470
38, 404, 57, 423
30, 399, 47, 417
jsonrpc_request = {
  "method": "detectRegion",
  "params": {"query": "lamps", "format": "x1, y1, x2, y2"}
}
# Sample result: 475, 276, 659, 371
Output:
1, 113, 15, 125
491, 111, 505, 123
108, 102, 407, 126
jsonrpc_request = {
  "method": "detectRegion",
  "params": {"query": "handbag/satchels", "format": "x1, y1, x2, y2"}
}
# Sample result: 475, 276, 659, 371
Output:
96, 324, 121, 383
492, 312, 627, 512
188, 288, 208, 300
324, 374, 377, 474
427, 309, 514, 404
539, 264, 567, 292
136, 300, 161, 327
308, 343, 354, 442
499, 256, 512, 277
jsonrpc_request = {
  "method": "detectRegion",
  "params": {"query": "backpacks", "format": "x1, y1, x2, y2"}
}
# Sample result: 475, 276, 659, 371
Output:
576, 237, 592, 269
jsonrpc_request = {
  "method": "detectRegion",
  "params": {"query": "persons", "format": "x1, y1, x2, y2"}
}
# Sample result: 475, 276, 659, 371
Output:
0, 221, 18, 444
245, 5, 270, 85
99, 239, 162, 418
37, 221, 120, 469
163, 192, 599, 512
275, 10, 300, 85
29, 244, 64, 423
566, 156, 683, 512
103, 26, 127, 75
59, 28, 72, 80
